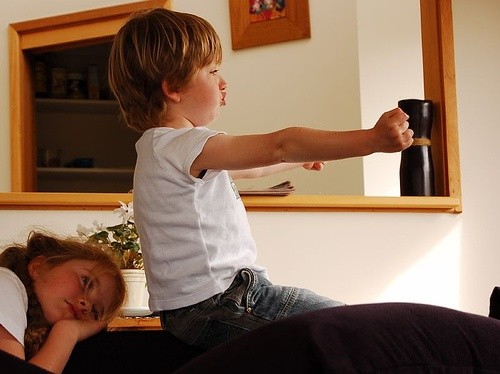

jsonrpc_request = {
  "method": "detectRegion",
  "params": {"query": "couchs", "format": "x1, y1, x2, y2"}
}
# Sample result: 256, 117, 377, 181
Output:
177, 302, 500, 374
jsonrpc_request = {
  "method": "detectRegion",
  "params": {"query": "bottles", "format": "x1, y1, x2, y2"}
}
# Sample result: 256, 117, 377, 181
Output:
88, 64, 100, 100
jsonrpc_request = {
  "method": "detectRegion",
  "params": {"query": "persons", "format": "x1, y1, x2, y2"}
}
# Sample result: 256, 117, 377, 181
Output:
0, 225, 128, 374
107, 8, 500, 349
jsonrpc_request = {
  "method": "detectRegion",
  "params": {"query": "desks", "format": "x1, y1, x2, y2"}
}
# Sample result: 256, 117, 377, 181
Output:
107, 316, 163, 331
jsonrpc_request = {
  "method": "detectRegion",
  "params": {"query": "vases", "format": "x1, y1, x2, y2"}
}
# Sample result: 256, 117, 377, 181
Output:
121, 270, 153, 316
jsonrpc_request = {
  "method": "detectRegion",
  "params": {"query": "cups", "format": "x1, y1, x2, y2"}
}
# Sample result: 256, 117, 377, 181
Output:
398, 99, 435, 196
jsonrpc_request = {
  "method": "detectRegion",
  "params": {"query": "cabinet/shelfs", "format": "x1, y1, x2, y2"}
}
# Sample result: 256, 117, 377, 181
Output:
34, 99, 134, 177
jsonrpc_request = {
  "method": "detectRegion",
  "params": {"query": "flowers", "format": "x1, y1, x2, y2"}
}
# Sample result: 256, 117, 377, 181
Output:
69, 200, 144, 270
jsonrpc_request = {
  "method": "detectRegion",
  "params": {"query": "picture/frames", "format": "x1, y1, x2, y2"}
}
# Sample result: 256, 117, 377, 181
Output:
228, 0, 311, 50
